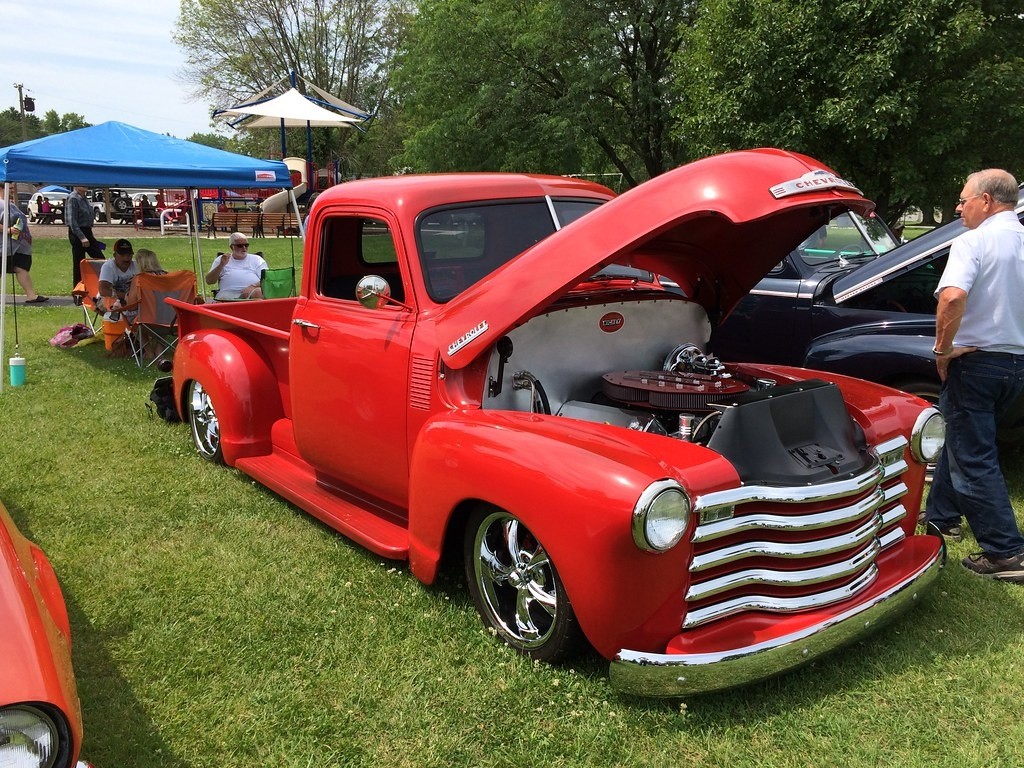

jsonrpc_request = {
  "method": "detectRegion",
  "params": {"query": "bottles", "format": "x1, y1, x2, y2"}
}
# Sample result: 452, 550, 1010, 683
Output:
110, 299, 121, 321
11, 217, 23, 240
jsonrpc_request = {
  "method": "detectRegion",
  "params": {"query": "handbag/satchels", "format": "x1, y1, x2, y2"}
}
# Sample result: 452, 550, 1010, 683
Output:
110, 332, 146, 358
50, 323, 94, 349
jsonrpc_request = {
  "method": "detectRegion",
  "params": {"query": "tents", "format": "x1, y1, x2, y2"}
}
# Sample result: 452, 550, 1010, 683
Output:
0, 121, 304, 395
38, 185, 72, 194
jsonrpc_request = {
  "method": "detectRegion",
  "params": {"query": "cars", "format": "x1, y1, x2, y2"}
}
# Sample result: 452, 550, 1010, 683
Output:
0, 497, 85, 768
11, 185, 247, 225
163, 145, 1024, 698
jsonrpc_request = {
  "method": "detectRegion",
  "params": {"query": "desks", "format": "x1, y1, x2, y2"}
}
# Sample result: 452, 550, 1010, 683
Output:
120, 206, 158, 224
51, 206, 65, 225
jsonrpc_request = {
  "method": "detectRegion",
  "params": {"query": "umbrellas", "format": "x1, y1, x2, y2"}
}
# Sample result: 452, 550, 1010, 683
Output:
212, 71, 372, 193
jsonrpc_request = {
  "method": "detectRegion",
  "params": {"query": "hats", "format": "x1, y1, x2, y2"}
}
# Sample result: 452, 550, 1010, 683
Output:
113, 239, 134, 254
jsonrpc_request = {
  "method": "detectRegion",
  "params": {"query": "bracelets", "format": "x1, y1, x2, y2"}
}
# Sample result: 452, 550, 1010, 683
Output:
251, 284, 258, 287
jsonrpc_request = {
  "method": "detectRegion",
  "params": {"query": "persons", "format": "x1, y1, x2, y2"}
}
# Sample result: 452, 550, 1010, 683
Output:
124, 249, 171, 362
37, 196, 66, 225
64, 186, 106, 307
0, 181, 49, 303
140, 195, 165, 220
96, 238, 142, 357
918, 169, 1024, 580
889, 222, 906, 247
799, 225, 826, 256
206, 232, 269, 303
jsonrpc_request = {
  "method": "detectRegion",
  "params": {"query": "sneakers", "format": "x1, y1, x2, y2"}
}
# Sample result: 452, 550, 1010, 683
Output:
962, 552, 1024, 580
918, 510, 962, 541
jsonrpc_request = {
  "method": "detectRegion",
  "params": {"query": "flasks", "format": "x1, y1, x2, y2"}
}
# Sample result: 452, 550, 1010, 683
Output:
9, 358, 26, 387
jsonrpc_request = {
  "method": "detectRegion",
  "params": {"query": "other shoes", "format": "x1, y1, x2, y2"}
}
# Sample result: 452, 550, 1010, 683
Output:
74, 296, 82, 305
157, 359, 171, 372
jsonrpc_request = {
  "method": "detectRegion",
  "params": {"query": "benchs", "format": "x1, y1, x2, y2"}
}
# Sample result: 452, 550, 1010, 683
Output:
116, 213, 160, 222
34, 213, 53, 224
205, 213, 305, 239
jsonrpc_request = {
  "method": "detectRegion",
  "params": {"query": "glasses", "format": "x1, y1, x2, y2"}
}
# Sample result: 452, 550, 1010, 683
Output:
959, 194, 982, 204
231, 243, 249, 248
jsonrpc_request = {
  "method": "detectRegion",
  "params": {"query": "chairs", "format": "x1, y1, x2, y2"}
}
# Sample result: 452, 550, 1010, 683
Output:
72, 251, 297, 373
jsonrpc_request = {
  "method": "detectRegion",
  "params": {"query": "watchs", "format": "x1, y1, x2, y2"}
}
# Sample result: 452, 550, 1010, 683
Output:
932, 342, 953, 356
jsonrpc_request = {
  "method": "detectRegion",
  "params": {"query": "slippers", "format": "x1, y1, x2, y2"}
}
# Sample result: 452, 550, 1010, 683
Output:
25, 296, 49, 303
